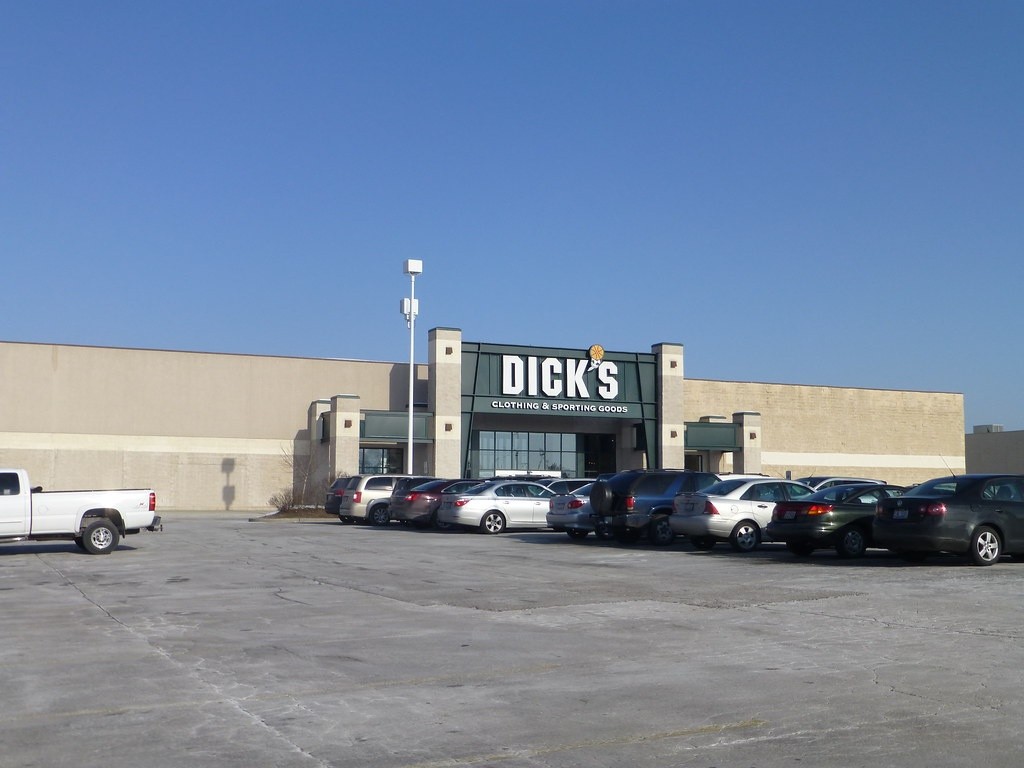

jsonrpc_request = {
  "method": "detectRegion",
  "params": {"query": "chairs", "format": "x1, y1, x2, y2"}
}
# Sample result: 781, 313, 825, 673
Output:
997, 486, 1012, 499
773, 488, 783, 501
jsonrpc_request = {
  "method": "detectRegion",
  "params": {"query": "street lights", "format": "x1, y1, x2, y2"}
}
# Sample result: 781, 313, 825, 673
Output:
400, 259, 423, 476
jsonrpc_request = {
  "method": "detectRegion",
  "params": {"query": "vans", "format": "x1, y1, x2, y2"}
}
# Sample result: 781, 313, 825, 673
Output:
533, 478, 603, 500
781, 477, 886, 499
392, 477, 438, 493
339, 474, 432, 525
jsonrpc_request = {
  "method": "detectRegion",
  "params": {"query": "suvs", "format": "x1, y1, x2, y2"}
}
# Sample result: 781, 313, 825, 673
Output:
590, 468, 723, 546
325, 477, 359, 523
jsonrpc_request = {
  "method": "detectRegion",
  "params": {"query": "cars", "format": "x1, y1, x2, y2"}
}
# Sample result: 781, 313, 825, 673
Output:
766, 483, 920, 558
873, 474, 1024, 566
545, 482, 613, 541
436, 480, 562, 534
389, 480, 494, 530
668, 478, 819, 552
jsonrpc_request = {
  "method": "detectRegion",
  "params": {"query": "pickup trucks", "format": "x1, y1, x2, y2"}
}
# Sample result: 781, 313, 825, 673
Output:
0, 469, 162, 555
